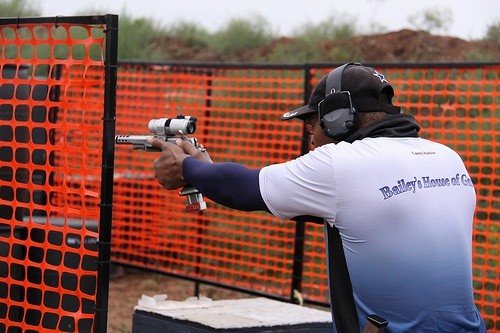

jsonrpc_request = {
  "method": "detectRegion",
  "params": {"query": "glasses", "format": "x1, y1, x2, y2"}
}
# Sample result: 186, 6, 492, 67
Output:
305, 115, 318, 135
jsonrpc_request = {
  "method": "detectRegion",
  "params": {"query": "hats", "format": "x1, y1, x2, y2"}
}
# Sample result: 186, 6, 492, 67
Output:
280, 64, 400, 121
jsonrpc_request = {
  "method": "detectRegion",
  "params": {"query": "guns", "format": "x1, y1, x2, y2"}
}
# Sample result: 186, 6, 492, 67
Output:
111, 113, 207, 213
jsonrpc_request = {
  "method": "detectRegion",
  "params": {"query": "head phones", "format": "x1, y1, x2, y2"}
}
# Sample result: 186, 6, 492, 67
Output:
318, 62, 359, 140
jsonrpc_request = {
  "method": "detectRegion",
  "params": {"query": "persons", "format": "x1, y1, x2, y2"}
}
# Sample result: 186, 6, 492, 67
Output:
146, 62, 487, 333
